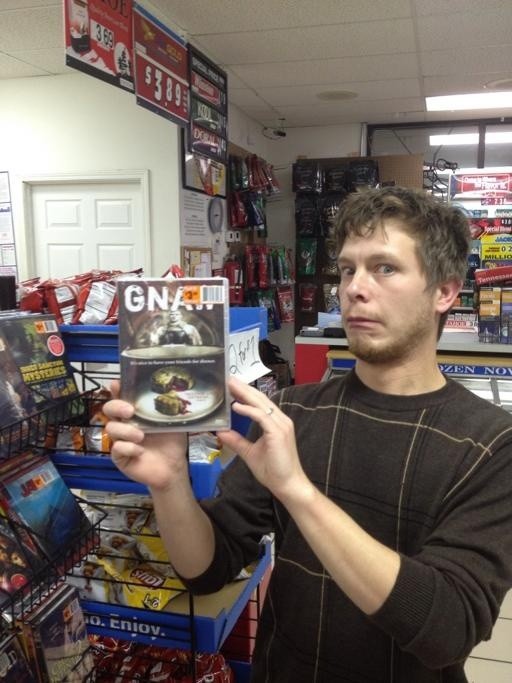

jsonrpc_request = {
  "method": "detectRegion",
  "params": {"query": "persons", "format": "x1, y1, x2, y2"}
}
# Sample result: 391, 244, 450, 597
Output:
148, 310, 203, 346
103, 185, 512, 683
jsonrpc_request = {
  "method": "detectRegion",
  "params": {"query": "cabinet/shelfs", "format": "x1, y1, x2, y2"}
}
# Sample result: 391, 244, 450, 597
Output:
294, 326, 511, 420
1, 325, 264, 683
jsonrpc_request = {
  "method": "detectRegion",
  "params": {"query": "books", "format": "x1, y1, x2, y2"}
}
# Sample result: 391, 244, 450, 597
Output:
117, 276, 233, 433
0, 308, 100, 683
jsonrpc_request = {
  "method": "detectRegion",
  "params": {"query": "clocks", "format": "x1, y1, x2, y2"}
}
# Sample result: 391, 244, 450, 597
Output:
209, 197, 228, 233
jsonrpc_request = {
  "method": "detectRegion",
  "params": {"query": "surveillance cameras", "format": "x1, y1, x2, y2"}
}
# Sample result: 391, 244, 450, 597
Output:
273, 129, 286, 137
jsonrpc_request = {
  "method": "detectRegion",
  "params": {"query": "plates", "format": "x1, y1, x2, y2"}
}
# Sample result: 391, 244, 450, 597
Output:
130, 372, 227, 425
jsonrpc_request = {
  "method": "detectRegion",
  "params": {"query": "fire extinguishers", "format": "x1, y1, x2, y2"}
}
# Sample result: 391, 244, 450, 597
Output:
223, 254, 244, 306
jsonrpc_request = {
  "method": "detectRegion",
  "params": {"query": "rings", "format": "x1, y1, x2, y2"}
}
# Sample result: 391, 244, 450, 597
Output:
266, 407, 273, 415
